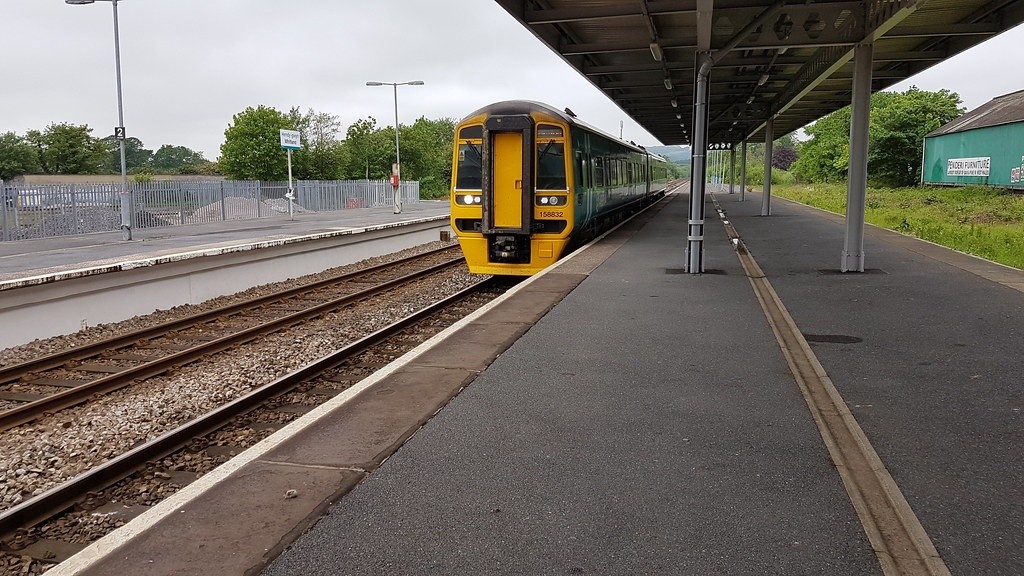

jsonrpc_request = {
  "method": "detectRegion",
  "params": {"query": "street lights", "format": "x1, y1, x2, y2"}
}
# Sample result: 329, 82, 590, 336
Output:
64, 0, 133, 243
365, 79, 426, 211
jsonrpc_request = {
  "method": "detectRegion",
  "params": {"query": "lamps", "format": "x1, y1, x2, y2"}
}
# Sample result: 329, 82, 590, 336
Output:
777, 47, 788, 54
671, 100, 677, 107
649, 43, 664, 62
676, 112, 741, 145
746, 96, 755, 104
664, 79, 673, 90
757, 75, 769, 86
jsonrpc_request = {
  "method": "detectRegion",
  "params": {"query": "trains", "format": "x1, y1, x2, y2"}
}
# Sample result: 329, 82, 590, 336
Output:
451, 99, 667, 277
